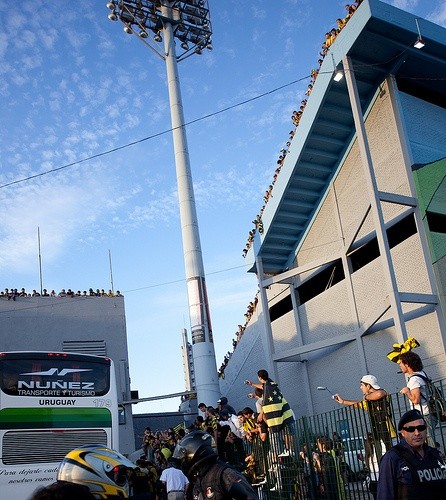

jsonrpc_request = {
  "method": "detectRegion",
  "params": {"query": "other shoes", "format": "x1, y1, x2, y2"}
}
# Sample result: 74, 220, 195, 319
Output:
269, 484, 283, 491
277, 449, 296, 457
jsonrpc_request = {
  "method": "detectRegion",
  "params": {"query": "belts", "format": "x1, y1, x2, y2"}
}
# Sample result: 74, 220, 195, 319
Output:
168, 490, 183, 494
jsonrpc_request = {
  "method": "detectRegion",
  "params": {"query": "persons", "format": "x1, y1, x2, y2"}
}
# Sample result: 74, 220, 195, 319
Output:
247, 369, 296, 457
0, 288, 123, 297
358, 433, 380, 500
242, 0, 364, 258
336, 375, 399, 459
216, 271, 277, 379
29, 388, 349, 500
400, 351, 438, 449
377, 410, 446, 500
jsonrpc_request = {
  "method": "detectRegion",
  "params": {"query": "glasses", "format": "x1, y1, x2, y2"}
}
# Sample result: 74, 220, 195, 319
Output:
402, 424, 426, 433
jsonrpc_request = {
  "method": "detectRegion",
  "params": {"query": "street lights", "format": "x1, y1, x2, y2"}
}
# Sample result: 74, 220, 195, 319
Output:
106, 0, 221, 417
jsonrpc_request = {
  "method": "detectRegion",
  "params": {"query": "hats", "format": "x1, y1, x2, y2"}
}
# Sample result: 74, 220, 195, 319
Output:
398, 409, 423, 430
361, 374, 381, 389
217, 397, 228, 403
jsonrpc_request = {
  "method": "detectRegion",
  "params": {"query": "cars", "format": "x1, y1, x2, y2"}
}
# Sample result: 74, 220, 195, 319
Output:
341, 436, 368, 481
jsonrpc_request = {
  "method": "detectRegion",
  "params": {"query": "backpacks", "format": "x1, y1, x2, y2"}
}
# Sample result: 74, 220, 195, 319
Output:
407, 370, 446, 422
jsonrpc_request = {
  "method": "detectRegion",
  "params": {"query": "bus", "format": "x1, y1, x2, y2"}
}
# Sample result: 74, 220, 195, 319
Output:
0, 350, 127, 500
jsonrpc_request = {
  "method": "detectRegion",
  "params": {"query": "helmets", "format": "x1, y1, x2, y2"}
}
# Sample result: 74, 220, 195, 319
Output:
177, 430, 217, 475
55, 443, 139, 499
